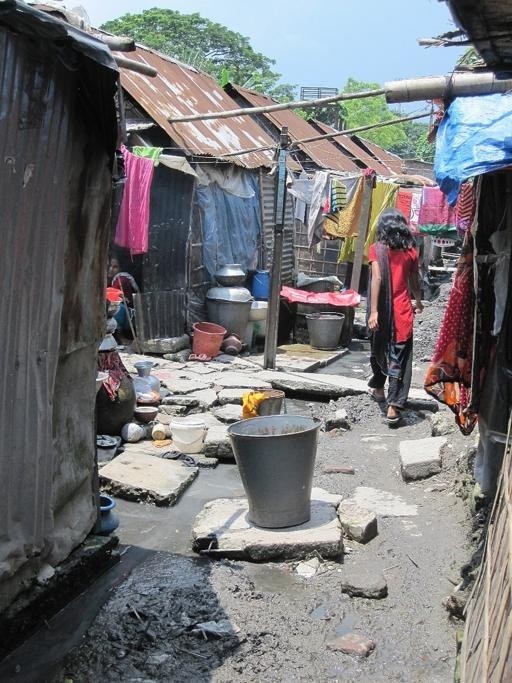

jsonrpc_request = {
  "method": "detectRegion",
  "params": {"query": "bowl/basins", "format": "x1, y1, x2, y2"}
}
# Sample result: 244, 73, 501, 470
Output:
135, 406, 157, 424
105, 286, 123, 302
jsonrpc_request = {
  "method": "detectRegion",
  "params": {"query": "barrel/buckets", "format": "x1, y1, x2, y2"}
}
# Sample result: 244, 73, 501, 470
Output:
305, 312, 344, 350
192, 321, 225, 358
253, 271, 271, 301
255, 388, 287, 416
168, 417, 206, 455
227, 415, 323, 529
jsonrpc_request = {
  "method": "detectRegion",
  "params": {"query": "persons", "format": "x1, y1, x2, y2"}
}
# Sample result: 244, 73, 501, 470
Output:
106, 250, 136, 349
361, 205, 425, 425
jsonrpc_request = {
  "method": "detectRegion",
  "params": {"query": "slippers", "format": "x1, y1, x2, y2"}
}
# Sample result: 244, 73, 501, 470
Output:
367, 387, 384, 402
386, 410, 402, 424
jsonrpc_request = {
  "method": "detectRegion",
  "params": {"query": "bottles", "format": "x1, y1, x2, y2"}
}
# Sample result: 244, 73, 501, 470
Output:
222, 333, 242, 353
96, 496, 121, 537
131, 361, 161, 403
